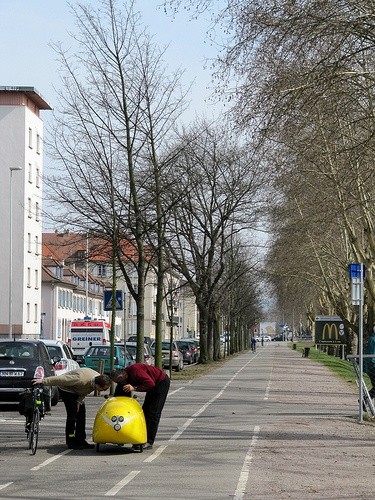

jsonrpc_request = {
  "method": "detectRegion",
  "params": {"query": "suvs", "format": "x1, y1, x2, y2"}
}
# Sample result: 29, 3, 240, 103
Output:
126, 336, 152, 346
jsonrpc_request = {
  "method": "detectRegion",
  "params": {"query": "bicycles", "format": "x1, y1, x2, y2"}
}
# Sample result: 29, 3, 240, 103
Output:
15, 379, 51, 456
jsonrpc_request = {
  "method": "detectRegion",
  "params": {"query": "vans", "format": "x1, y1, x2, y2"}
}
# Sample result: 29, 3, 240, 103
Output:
69, 320, 118, 362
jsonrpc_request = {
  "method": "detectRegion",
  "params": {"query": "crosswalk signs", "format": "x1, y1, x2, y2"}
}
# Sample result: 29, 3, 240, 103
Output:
102, 290, 123, 311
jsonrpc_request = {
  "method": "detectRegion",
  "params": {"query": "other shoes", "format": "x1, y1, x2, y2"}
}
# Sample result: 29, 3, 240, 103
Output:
78, 440, 94, 449
68, 440, 84, 449
131, 444, 141, 453
143, 443, 153, 449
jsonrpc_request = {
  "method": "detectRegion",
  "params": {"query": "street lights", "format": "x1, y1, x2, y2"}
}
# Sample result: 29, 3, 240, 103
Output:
9, 165, 23, 340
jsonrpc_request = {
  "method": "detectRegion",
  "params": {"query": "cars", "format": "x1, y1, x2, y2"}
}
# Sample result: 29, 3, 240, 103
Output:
37, 339, 85, 376
271, 334, 289, 342
175, 340, 200, 365
0, 339, 59, 410
150, 341, 184, 372
255, 336, 272, 342
78, 346, 136, 377
113, 342, 156, 368
219, 335, 225, 342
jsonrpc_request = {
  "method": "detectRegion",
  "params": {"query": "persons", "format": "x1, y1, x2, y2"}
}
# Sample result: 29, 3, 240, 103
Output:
358, 321, 375, 412
261, 337, 264, 346
32, 367, 111, 449
110, 362, 170, 450
251, 338, 256, 352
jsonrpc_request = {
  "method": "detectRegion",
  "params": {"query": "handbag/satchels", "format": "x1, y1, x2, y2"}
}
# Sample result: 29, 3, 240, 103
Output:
18, 392, 51, 415
